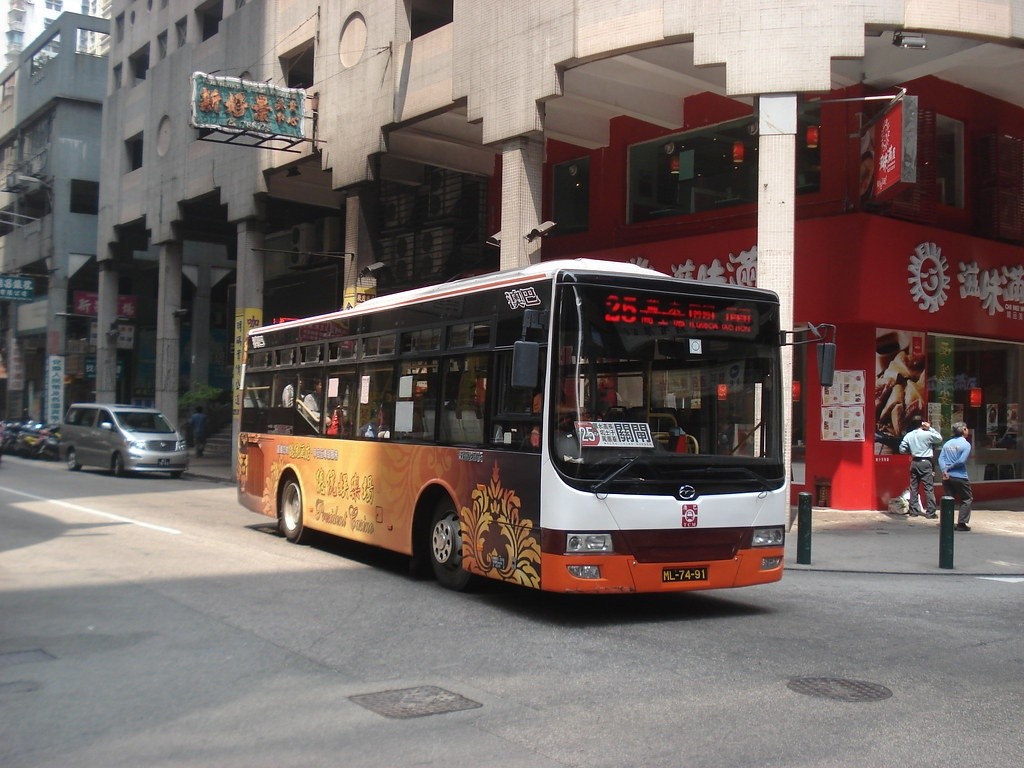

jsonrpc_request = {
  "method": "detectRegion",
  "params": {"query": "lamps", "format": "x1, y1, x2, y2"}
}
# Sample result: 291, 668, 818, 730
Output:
110, 318, 130, 331
523, 221, 557, 242
486, 232, 501, 247
359, 262, 386, 279
172, 308, 188, 317
892, 30, 929, 51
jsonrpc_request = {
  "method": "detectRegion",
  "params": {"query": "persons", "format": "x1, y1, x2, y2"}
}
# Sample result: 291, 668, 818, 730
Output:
280, 371, 332, 433
898, 416, 943, 518
186, 404, 210, 459
937, 422, 973, 532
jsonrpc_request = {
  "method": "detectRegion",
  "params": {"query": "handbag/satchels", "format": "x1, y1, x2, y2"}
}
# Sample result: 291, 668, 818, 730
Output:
888, 496, 910, 515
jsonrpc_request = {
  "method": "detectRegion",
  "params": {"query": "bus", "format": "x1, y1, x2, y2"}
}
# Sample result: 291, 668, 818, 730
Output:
239, 258, 836, 593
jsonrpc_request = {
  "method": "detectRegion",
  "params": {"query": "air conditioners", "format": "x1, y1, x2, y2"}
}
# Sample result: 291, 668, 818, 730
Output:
375, 166, 460, 276
289, 223, 313, 269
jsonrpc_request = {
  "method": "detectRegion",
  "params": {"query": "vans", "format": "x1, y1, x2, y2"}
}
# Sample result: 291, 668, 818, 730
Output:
59, 402, 189, 478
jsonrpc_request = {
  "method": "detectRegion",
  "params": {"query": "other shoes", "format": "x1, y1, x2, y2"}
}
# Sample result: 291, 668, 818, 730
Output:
956, 523, 971, 531
928, 514, 938, 519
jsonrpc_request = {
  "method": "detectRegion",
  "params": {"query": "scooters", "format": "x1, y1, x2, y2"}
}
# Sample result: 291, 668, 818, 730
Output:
0, 419, 60, 460
